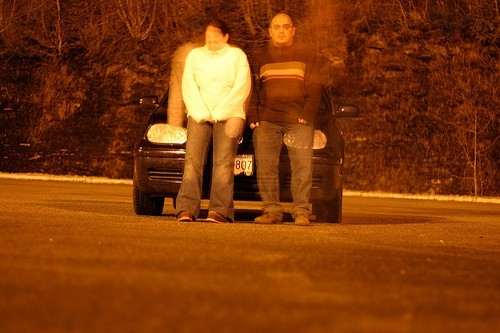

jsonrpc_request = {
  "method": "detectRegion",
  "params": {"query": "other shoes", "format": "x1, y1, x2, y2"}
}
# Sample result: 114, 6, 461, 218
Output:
206, 212, 229, 223
254, 212, 282, 224
177, 211, 193, 221
295, 215, 309, 226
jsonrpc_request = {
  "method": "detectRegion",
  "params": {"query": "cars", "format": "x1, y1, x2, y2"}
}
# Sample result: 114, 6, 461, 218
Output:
132, 79, 361, 223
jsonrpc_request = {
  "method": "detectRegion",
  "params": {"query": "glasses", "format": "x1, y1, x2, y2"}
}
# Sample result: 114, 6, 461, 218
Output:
270, 25, 293, 30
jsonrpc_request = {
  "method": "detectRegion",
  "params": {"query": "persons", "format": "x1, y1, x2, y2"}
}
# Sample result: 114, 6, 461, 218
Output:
174, 18, 252, 221
248, 12, 322, 224
